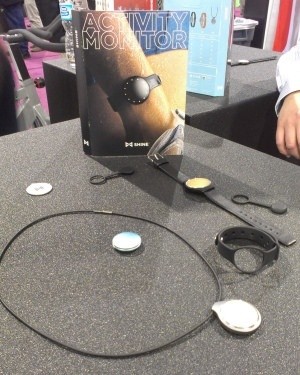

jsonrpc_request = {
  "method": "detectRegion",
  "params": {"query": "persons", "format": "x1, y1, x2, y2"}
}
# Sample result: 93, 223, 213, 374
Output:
78, 11, 182, 156
273, 31, 300, 162
0, 0, 66, 58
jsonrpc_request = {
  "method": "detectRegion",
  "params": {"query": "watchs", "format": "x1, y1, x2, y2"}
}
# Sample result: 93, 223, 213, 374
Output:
231, 54, 278, 68
148, 150, 298, 247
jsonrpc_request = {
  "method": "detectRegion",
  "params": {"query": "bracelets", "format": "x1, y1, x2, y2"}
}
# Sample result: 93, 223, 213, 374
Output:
213, 223, 283, 277
106, 71, 162, 113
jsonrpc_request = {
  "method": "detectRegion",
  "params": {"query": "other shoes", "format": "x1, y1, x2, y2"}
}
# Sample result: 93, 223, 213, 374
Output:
29, 43, 45, 53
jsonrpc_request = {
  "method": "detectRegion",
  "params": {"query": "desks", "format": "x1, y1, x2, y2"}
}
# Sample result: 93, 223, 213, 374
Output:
42, 44, 300, 166
0, 118, 300, 375
